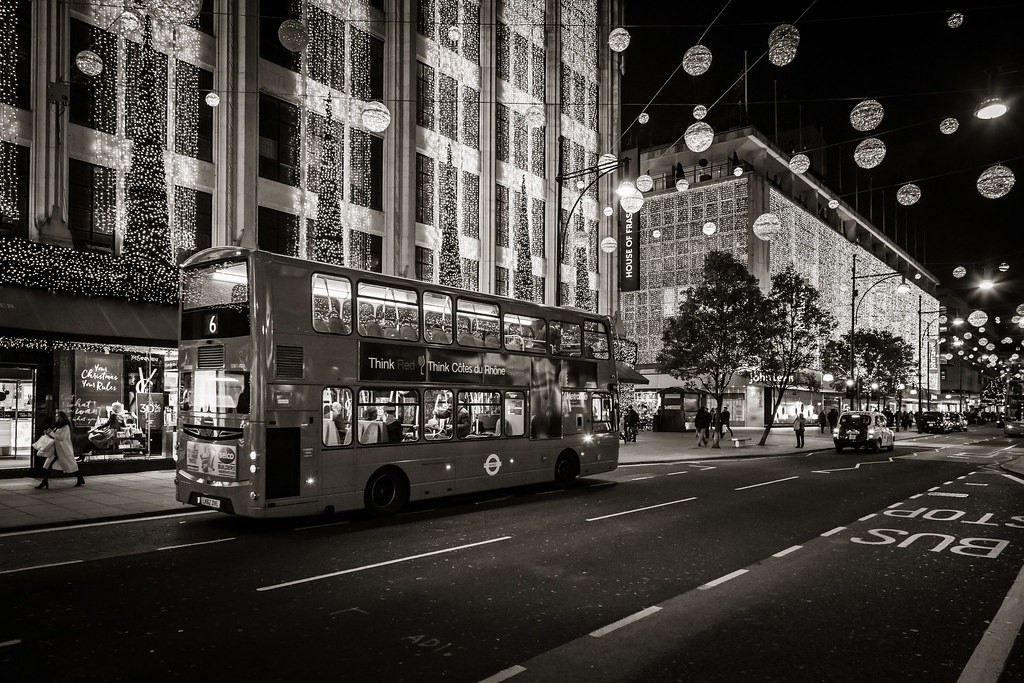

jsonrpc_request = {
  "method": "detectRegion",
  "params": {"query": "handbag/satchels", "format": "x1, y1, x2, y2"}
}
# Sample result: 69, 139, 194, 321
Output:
721, 425, 726, 433
793, 420, 800, 430
32, 434, 55, 458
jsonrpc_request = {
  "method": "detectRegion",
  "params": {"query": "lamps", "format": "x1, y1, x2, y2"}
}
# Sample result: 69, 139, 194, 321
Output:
277, 19, 309, 51
448, 22, 461, 41
76, 50, 103, 75
527, 0, 1024, 410
205, 92, 220, 106
121, 7, 146, 32
361, 99, 392, 135
141, 0, 202, 24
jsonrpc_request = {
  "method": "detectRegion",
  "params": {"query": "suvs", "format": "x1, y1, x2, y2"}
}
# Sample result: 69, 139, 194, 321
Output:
832, 410, 896, 453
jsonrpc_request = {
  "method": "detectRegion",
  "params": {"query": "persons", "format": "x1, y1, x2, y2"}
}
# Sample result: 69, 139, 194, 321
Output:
35, 411, 85, 489
363, 406, 406, 442
623, 406, 637, 442
793, 414, 806, 448
432, 397, 471, 438
325, 403, 347, 434
76, 402, 125, 461
532, 322, 579, 351
818, 409, 838, 433
881, 409, 923, 430
694, 406, 734, 446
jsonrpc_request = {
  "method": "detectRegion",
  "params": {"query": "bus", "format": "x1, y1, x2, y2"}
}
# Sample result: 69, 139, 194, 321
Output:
171, 243, 621, 528
1000, 360, 1024, 436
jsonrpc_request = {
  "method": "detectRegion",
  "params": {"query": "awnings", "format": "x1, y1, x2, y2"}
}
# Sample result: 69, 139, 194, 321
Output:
616, 362, 649, 384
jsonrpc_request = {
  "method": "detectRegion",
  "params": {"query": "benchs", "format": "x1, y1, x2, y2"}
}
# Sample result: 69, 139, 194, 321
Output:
726, 436, 751, 447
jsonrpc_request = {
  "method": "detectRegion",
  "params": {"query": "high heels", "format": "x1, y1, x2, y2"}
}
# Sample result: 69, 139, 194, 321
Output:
75, 478, 85, 487
35, 480, 48, 489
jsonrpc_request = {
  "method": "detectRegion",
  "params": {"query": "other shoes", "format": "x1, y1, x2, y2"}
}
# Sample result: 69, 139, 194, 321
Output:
632, 440, 635, 442
796, 445, 800, 448
802, 444, 804, 448
698, 443, 702, 446
705, 441, 708, 446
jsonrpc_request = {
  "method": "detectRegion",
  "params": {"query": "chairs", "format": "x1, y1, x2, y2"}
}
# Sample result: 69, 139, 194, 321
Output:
314, 311, 525, 352
321, 414, 498, 445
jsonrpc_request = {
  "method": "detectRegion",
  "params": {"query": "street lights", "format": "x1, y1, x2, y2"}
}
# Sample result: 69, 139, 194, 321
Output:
916, 293, 967, 435
848, 253, 912, 413
552, 134, 639, 307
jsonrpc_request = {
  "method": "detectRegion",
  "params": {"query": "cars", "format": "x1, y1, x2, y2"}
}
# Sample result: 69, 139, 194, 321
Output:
916, 410, 968, 434
